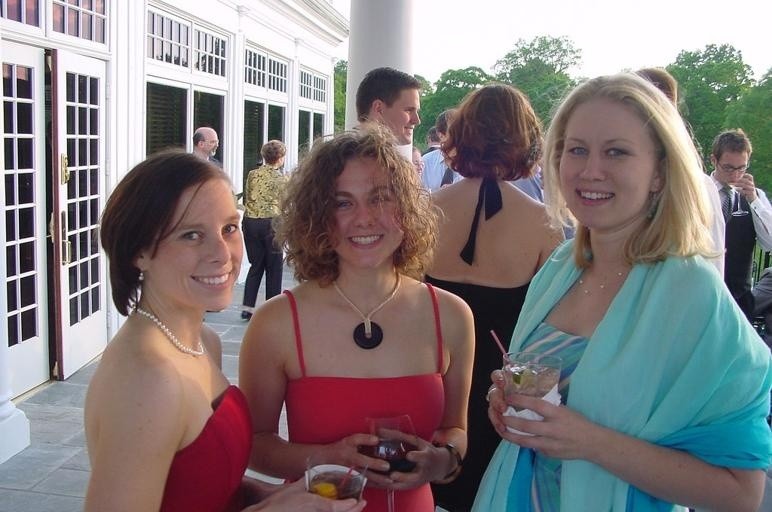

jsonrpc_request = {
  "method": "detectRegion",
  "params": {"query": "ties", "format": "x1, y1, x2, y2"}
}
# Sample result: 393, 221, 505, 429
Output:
719, 186, 733, 224
440, 167, 453, 189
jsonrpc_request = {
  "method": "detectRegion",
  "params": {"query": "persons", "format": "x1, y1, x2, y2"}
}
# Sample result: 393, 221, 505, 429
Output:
510, 166, 576, 240
236, 123, 477, 512
411, 147, 425, 177
472, 71, 771, 511
420, 126, 444, 157
355, 67, 422, 147
192, 126, 223, 168
423, 84, 567, 440
635, 68, 677, 110
241, 139, 291, 322
84, 151, 254, 512
421, 109, 464, 194
711, 129, 772, 322
751, 265, 772, 348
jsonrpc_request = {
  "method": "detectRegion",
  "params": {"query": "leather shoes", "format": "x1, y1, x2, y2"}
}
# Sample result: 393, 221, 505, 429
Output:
241, 311, 252, 320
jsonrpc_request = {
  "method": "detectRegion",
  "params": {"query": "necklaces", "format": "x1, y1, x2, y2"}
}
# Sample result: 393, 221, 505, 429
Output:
331, 267, 402, 349
133, 308, 205, 357
572, 262, 631, 295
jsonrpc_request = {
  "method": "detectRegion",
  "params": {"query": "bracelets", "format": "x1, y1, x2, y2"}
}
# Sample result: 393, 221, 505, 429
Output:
422, 437, 470, 490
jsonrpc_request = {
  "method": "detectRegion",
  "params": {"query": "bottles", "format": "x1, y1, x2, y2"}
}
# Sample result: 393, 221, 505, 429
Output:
754, 316, 765, 337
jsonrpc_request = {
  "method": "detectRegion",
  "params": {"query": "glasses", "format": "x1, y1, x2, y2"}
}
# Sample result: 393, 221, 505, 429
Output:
200, 139, 219, 145
717, 160, 749, 171
